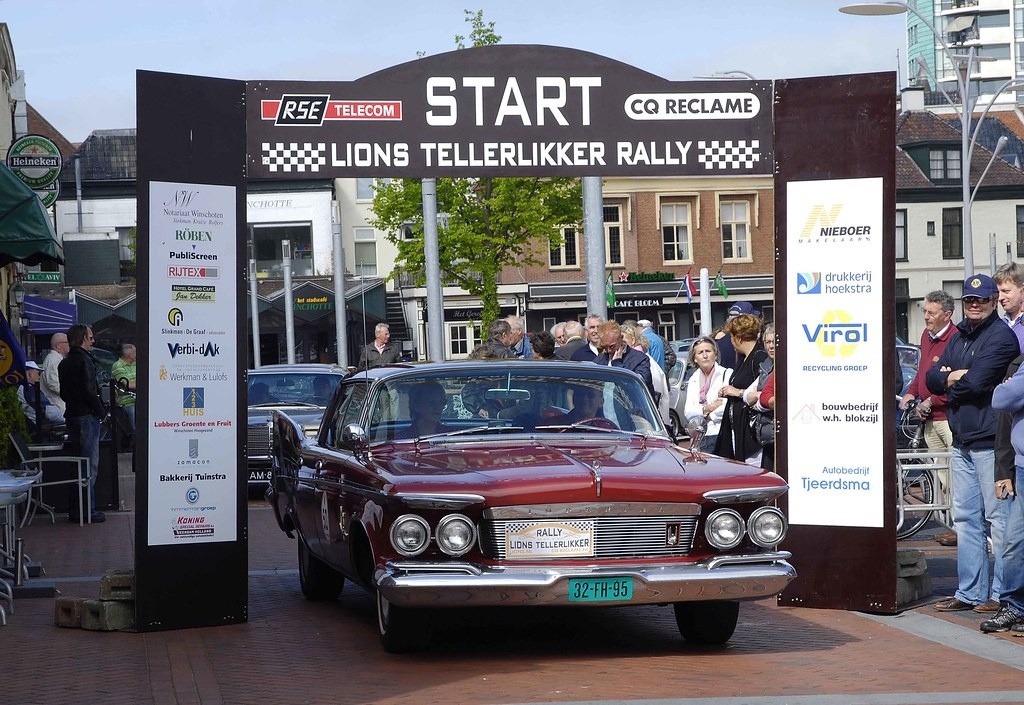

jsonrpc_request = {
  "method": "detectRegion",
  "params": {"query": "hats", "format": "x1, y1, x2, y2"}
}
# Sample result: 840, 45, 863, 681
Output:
727, 302, 761, 317
637, 319, 652, 327
25, 361, 44, 371
961, 273, 999, 299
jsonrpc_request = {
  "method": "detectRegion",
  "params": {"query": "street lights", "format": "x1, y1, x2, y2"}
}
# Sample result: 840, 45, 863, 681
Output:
838, 3, 1024, 280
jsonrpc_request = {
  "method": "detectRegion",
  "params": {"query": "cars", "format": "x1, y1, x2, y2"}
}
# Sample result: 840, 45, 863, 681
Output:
667, 338, 697, 438
89, 348, 134, 452
896, 344, 921, 439
247, 364, 358, 486
268, 361, 797, 646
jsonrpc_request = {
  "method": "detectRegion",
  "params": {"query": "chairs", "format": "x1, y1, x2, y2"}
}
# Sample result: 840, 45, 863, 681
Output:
8, 433, 92, 528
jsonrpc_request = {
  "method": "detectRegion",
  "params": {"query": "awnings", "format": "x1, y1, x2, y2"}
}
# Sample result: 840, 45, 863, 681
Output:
0, 160, 66, 266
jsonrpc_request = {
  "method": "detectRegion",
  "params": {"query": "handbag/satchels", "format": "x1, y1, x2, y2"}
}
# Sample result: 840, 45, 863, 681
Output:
742, 350, 770, 440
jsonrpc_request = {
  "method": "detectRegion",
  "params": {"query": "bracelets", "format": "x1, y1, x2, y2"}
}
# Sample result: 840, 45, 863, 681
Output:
927, 397, 934, 406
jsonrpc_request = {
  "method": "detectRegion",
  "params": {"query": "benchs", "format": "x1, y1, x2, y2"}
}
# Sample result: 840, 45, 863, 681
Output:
368, 421, 514, 441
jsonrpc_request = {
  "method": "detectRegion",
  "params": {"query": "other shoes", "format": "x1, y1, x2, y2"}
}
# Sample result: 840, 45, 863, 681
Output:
941, 534, 958, 546
25, 487, 121, 524
973, 599, 1001, 613
931, 597, 977, 612
933, 530, 953, 541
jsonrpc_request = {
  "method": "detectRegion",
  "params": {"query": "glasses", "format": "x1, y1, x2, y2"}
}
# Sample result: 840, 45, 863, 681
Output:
692, 339, 715, 348
483, 354, 498, 359
574, 389, 602, 399
963, 296, 997, 304
90, 336, 95, 340
415, 396, 448, 407
601, 335, 619, 349
920, 307, 946, 318
763, 339, 774, 346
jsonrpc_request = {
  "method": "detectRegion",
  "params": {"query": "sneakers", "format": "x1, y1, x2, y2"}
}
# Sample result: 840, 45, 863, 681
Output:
979, 600, 1024, 632
1010, 615, 1024, 636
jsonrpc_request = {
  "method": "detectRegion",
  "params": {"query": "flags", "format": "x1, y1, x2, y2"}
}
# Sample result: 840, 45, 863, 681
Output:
605, 269, 614, 309
713, 272, 728, 300
683, 272, 698, 305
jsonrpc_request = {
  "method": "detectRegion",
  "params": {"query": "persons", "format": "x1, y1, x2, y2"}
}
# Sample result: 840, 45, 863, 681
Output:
358, 323, 402, 372
926, 273, 1019, 610
979, 360, 1024, 636
898, 290, 960, 546
248, 382, 279, 406
388, 379, 476, 441
461, 314, 677, 430
42, 333, 71, 416
111, 344, 136, 430
312, 376, 334, 405
992, 353, 1024, 499
992, 261, 1024, 351
17, 359, 65, 436
57, 323, 107, 522
684, 300, 904, 473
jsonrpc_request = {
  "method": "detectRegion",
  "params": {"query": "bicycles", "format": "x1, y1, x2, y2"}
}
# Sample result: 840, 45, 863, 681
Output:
893, 393, 934, 541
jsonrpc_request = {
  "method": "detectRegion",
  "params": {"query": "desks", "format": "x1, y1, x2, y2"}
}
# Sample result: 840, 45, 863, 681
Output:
0, 467, 44, 626
26, 442, 63, 525
